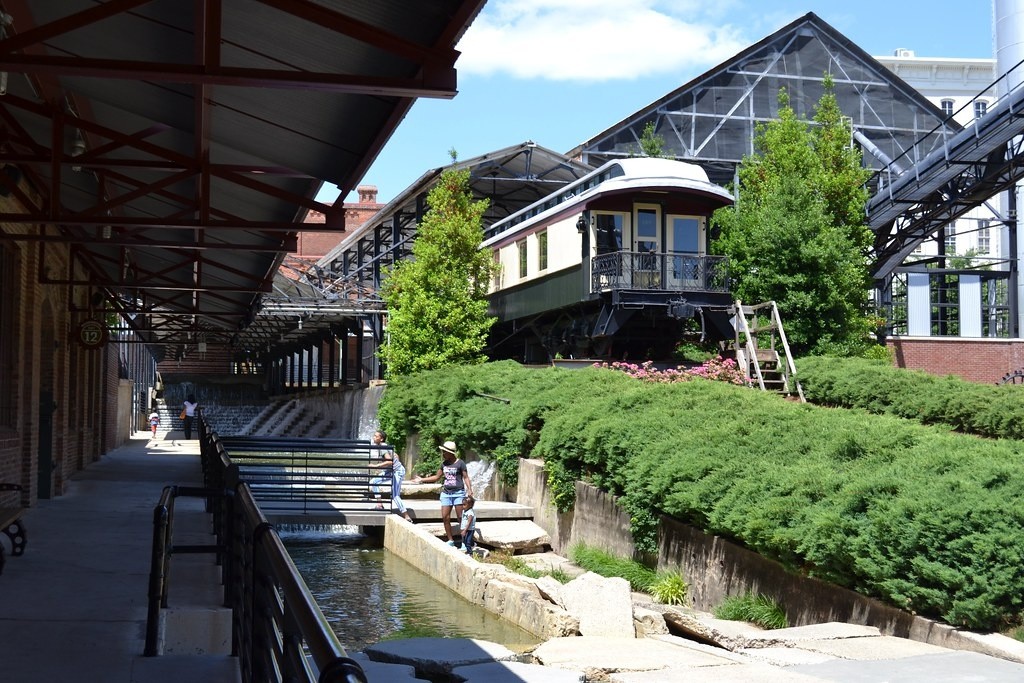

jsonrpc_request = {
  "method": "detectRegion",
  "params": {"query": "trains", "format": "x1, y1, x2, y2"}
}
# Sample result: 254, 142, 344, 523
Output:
462, 156, 735, 365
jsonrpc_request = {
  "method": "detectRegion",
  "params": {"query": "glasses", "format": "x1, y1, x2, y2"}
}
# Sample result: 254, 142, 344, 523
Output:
443, 450, 446, 453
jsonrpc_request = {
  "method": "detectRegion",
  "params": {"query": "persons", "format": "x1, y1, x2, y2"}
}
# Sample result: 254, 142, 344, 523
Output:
460, 495, 477, 554
411, 441, 474, 545
179, 394, 198, 439
367, 429, 413, 523
149, 407, 160, 439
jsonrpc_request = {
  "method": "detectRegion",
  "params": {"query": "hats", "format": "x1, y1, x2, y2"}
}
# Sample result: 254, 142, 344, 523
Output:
438, 441, 456, 455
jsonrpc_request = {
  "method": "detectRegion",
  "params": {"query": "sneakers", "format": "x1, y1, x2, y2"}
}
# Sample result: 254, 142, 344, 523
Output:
460, 542, 467, 551
444, 540, 454, 546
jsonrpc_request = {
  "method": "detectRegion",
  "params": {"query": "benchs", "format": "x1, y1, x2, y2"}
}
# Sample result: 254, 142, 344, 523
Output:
0, 483, 27, 575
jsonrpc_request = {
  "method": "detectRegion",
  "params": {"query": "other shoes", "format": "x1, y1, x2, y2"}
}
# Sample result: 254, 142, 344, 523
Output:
406, 518, 413, 523
370, 505, 384, 510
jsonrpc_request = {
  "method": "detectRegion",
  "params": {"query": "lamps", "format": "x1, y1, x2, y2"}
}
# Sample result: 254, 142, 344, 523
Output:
71, 140, 85, 171
104, 208, 113, 238
575, 216, 587, 234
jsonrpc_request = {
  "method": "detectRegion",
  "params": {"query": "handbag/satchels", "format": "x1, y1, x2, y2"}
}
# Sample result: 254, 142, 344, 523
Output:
180, 409, 186, 419
